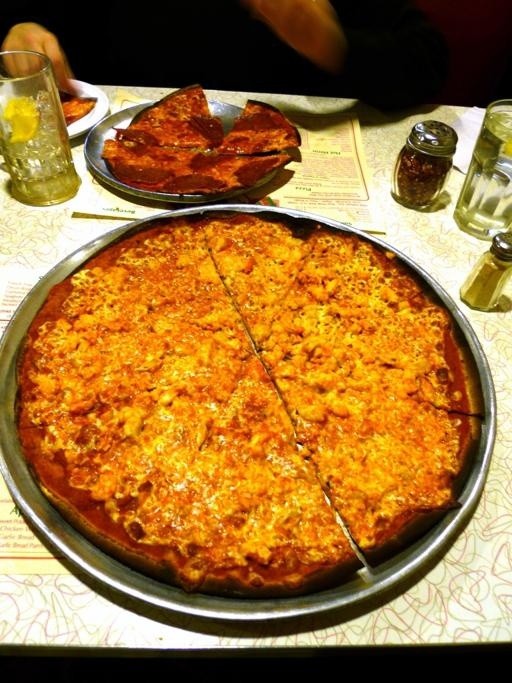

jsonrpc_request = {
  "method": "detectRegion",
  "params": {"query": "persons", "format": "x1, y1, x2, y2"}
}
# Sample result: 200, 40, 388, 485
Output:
0, 0, 348, 94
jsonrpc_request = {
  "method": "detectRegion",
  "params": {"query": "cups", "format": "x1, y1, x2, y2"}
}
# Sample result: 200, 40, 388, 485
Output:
453, 97, 511, 242
0, 50, 79, 207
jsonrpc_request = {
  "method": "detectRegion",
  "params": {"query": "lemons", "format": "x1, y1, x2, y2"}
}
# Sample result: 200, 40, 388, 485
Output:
1, 96, 39, 144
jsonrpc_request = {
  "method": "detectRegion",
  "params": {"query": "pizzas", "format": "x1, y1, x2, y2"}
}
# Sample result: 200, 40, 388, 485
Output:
98, 81, 300, 194
57, 91, 97, 127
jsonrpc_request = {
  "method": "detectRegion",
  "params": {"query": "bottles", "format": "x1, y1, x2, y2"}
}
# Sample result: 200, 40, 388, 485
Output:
459, 231, 511, 313
390, 120, 459, 211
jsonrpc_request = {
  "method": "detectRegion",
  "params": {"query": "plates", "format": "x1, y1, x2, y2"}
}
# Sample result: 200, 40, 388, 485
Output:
83, 97, 287, 205
1, 78, 110, 141
